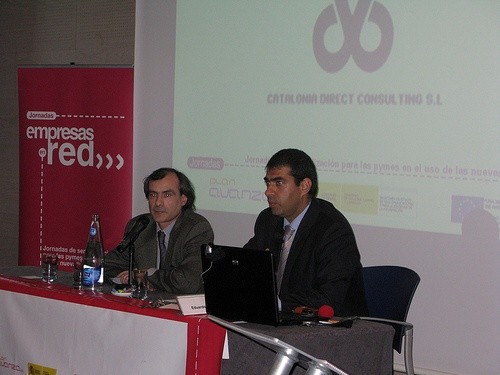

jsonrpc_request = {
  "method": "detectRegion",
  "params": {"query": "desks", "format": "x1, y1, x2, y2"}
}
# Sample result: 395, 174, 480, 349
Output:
0, 264, 396, 375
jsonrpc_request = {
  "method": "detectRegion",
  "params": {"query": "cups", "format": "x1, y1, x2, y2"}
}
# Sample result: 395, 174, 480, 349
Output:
73, 258, 84, 290
42, 254, 58, 288
131, 268, 148, 301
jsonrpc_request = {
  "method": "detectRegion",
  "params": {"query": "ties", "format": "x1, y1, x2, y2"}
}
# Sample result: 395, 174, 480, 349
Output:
158, 230, 167, 270
276, 225, 296, 293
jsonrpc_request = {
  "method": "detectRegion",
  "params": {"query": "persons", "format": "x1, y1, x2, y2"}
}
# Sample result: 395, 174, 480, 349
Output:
242, 148, 367, 319
104, 167, 215, 295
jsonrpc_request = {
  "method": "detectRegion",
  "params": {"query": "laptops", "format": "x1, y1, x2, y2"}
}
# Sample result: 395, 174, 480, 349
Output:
199, 244, 328, 326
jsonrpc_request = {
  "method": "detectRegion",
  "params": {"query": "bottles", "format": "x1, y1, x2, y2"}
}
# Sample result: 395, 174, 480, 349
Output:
82, 214, 104, 288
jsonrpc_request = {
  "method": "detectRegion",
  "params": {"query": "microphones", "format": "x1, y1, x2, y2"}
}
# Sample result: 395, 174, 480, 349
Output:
116, 215, 150, 252
303, 305, 334, 318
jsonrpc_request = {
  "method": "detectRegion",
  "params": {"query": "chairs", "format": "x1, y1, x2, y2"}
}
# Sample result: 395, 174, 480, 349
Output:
349, 265, 421, 375
